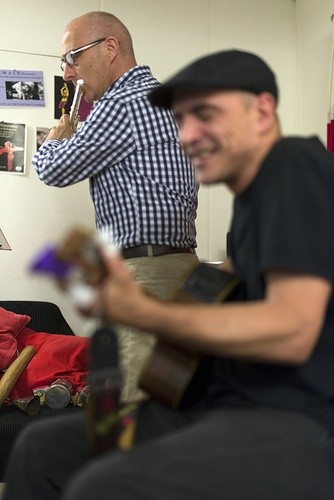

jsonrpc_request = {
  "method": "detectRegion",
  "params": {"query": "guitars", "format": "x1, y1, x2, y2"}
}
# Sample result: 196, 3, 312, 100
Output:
28, 232, 240, 415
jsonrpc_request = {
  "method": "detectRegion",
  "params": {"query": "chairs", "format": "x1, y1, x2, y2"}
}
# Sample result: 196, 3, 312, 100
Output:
0, 301, 87, 484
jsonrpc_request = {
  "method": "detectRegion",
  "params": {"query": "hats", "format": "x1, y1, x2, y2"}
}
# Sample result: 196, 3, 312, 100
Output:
146, 49, 279, 110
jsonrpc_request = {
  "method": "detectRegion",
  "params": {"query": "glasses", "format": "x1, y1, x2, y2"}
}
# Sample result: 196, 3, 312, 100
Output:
60, 37, 107, 71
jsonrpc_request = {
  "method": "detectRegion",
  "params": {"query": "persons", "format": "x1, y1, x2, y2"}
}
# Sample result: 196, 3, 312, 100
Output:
31, 11, 200, 401
4, 140, 15, 171
11, 81, 38, 99
0, 50, 334, 500
59, 83, 69, 115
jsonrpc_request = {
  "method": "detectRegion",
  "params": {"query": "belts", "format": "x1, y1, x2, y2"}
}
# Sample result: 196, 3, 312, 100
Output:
119, 243, 197, 261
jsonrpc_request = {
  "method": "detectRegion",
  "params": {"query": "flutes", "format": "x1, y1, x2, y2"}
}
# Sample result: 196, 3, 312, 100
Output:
68, 79, 85, 136
